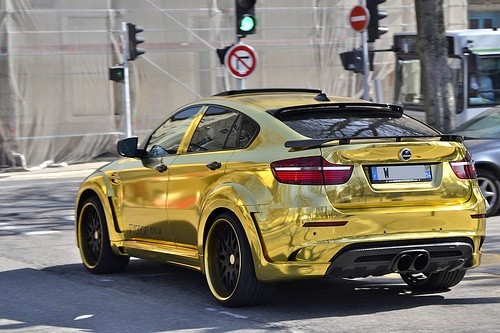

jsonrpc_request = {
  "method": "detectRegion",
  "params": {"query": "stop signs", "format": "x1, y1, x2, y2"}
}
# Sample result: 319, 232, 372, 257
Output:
349, 5, 370, 31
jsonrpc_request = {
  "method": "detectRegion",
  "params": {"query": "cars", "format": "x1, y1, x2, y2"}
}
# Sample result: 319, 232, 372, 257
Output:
443, 104, 500, 218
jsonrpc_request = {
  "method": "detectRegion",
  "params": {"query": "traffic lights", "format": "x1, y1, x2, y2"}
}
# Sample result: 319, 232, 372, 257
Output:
234, 0, 257, 35
367, 0, 390, 42
108, 67, 124, 82
127, 22, 146, 61
339, 50, 363, 73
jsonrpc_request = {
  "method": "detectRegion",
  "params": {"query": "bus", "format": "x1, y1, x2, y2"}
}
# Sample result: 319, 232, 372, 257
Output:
366, 26, 500, 132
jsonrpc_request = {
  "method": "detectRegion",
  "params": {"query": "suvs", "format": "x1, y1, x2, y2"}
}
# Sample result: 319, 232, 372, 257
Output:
75, 86, 488, 308
